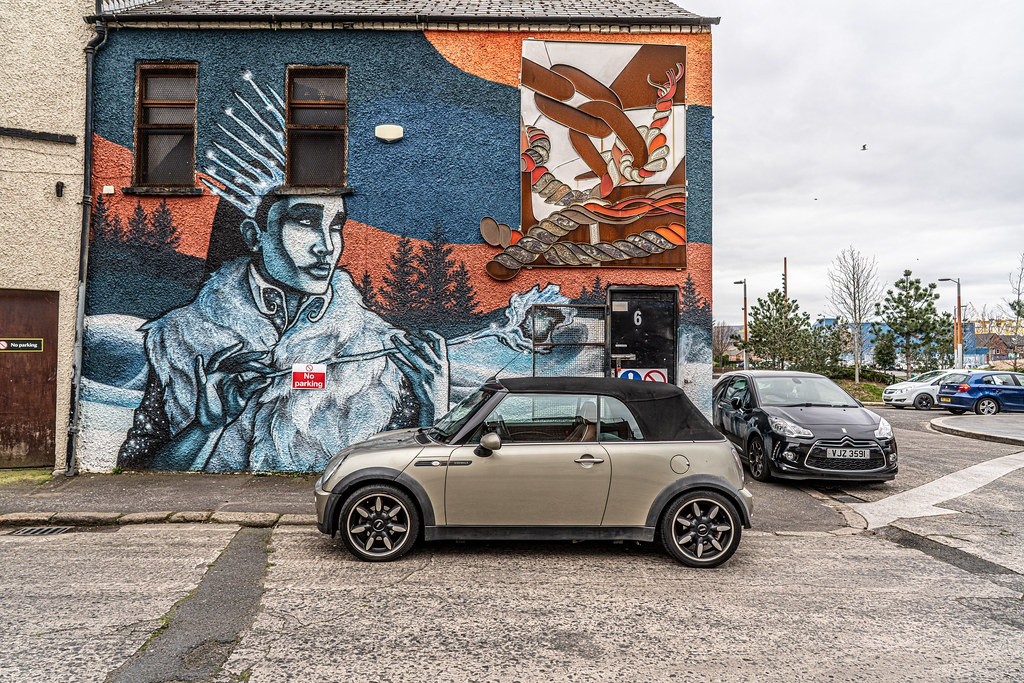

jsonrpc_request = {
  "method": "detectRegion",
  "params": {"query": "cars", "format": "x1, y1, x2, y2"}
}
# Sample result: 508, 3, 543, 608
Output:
736, 359, 790, 370
713, 371, 900, 486
312, 377, 755, 569
936, 370, 1024, 415
882, 370, 1003, 411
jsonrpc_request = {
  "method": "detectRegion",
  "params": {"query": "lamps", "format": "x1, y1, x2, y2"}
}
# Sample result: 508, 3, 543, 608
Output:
374, 125, 404, 140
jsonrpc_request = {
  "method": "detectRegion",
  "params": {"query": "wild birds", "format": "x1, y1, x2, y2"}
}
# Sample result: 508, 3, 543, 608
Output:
860, 143, 868, 151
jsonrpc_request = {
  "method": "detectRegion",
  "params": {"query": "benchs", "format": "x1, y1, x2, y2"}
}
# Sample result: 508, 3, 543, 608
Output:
759, 387, 796, 399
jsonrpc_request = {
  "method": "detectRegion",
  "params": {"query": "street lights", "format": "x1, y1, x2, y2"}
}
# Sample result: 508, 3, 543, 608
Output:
938, 278, 964, 368
733, 278, 747, 370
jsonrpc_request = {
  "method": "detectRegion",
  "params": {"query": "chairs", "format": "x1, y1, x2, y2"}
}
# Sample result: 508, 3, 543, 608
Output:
800, 385, 819, 402
563, 402, 596, 442
1003, 381, 1009, 385
770, 380, 794, 400
